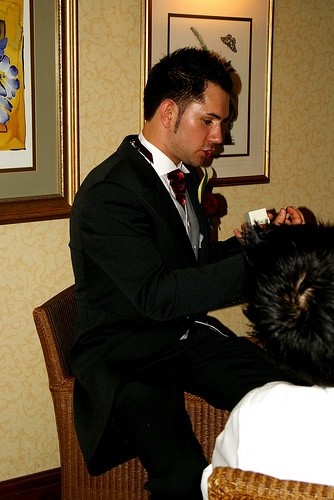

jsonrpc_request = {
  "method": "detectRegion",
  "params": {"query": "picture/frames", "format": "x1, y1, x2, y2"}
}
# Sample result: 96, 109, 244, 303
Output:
0, 0, 80, 227
138, 0, 274, 187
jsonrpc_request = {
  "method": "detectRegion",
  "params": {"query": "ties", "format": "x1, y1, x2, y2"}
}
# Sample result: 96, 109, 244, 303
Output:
168, 169, 187, 212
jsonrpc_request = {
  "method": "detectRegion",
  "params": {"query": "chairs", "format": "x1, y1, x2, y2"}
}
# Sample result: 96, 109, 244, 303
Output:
32, 283, 232, 500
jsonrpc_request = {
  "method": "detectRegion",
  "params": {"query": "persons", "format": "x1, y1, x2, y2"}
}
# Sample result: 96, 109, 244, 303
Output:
68, 48, 305, 500
199, 220, 334, 500
